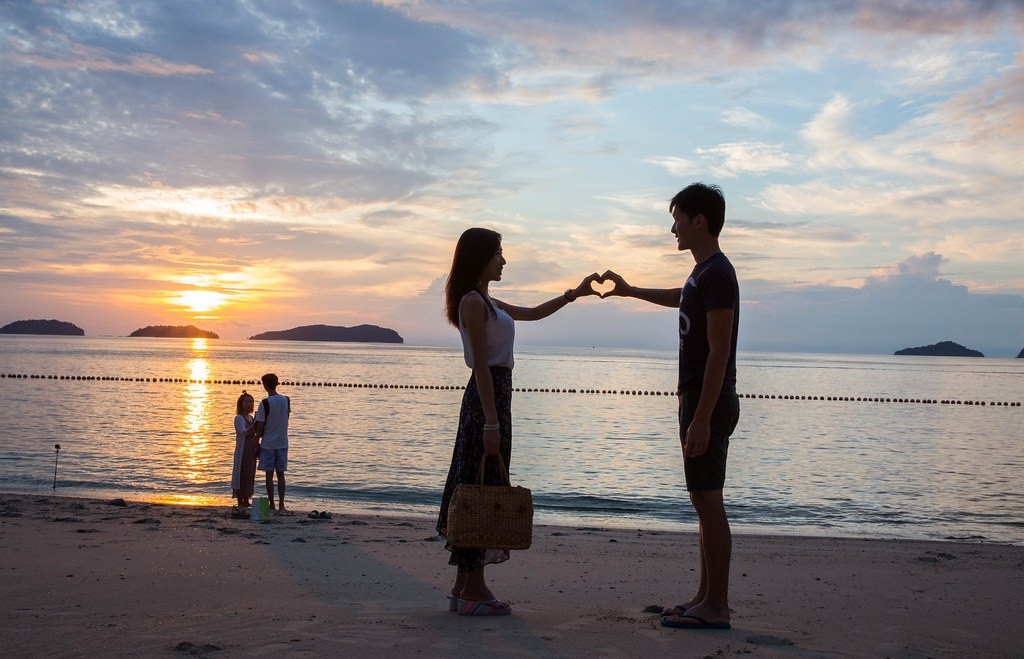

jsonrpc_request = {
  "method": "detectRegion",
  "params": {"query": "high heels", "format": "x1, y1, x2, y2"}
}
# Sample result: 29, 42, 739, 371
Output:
449, 597, 458, 611
457, 598, 511, 615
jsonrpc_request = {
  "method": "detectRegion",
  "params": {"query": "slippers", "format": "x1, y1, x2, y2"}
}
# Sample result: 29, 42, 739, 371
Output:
660, 605, 687, 617
660, 611, 732, 629
308, 510, 330, 519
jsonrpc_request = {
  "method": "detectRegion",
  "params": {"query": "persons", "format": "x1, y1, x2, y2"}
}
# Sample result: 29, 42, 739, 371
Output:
601, 184, 740, 628
231, 374, 296, 516
436, 228, 601, 615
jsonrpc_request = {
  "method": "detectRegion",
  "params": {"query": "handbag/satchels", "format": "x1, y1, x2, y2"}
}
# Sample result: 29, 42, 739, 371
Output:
447, 451, 533, 551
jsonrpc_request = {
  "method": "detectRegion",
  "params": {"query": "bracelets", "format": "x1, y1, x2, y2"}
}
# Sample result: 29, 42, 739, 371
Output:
565, 289, 576, 302
483, 423, 500, 430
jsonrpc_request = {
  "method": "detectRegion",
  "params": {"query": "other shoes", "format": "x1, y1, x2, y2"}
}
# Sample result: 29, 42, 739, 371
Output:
231, 506, 250, 519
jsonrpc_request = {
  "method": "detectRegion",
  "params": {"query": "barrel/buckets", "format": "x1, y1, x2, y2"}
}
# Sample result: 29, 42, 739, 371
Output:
252, 496, 270, 522
